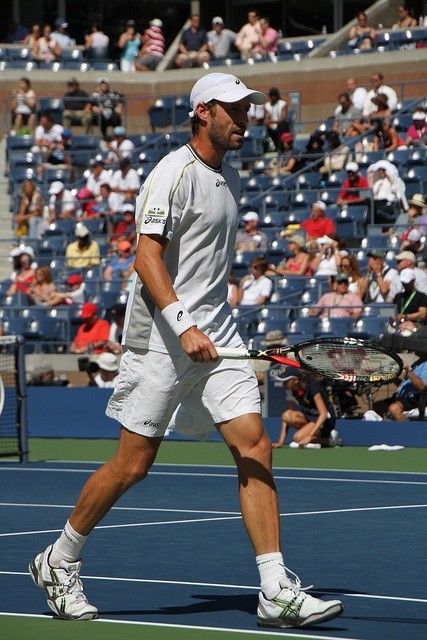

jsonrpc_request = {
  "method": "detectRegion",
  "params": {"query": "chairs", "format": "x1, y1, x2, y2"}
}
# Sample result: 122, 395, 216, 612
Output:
3, 134, 159, 229
316, 98, 426, 231
169, 31, 311, 68
146, 94, 195, 127
210, 232, 427, 354
15, 89, 122, 128
336, 25, 427, 54
1, 237, 136, 356
3, 41, 127, 76
162, 134, 318, 229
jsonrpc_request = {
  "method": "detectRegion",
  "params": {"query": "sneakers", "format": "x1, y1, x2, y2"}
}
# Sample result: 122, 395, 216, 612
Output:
331, 430, 342, 446
256, 577, 345, 630
27, 544, 99, 621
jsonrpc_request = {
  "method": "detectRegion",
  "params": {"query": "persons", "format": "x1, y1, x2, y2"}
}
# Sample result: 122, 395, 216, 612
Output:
237, 258, 273, 305
205, 16, 236, 60
398, 227, 427, 269
27, 265, 55, 302
226, 272, 238, 308
100, 183, 135, 234
366, 160, 410, 212
339, 255, 361, 293
345, 94, 391, 137
70, 303, 110, 354
277, 29, 283, 39
110, 203, 137, 246
61, 77, 93, 135
306, 273, 364, 321
50, 18, 76, 46
247, 18, 278, 60
325, 130, 353, 172
235, 11, 264, 60
31, 25, 61, 62
28, 73, 345, 626
262, 132, 303, 174
390, 193, 426, 235
6, 79, 37, 134
264, 86, 289, 136
271, 367, 336, 448
373, 118, 407, 151
381, 267, 427, 350
252, 330, 294, 385
103, 303, 126, 354
101, 126, 134, 165
120, 260, 137, 304
23, 24, 40, 45
175, 14, 210, 69
84, 23, 110, 49
85, 157, 111, 196
336, 162, 370, 205
48, 181, 76, 222
394, 321, 427, 356
89, 352, 121, 389
14, 179, 45, 239
265, 235, 313, 278
405, 110, 427, 148
244, 102, 266, 126
349, 11, 376, 50
30, 112, 71, 154
394, 251, 427, 295
85, 78, 123, 141
281, 200, 335, 250
112, 156, 140, 200
388, 351, 427, 421
26, 361, 71, 387
300, 133, 325, 172
360, 249, 401, 304
5, 245, 36, 296
36, 130, 78, 184
310, 234, 341, 276
103, 239, 133, 281
75, 187, 97, 217
135, 19, 163, 71
48, 274, 91, 306
233, 211, 267, 252
66, 225, 100, 269
396, 4, 417, 30
331, 92, 360, 137
118, 20, 141, 73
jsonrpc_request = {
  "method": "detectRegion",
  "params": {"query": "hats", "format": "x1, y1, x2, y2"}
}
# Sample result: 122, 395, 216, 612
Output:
48, 180, 64, 195
212, 16, 223, 26
121, 203, 135, 214
75, 188, 92, 199
400, 268, 417, 284
400, 228, 422, 242
97, 77, 109, 85
371, 94, 389, 109
317, 234, 334, 245
79, 300, 97, 319
66, 274, 82, 284
187, 72, 266, 119
345, 163, 359, 173
275, 366, 301, 381
285, 234, 305, 248
393, 250, 416, 264
408, 194, 427, 207
96, 351, 119, 372
113, 126, 127, 137
149, 19, 162, 26
109, 304, 125, 320
67, 78, 79, 86
336, 273, 348, 282
241, 211, 259, 223
366, 247, 386, 259
312, 200, 327, 212
75, 225, 89, 237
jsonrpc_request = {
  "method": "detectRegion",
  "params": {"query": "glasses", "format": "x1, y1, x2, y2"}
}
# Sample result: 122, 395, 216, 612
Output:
249, 265, 260, 270
340, 265, 351, 269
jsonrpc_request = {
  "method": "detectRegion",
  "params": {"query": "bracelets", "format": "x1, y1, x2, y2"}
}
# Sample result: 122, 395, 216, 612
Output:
374, 274, 382, 278
307, 433, 313, 437
159, 300, 198, 339
366, 273, 369, 276
